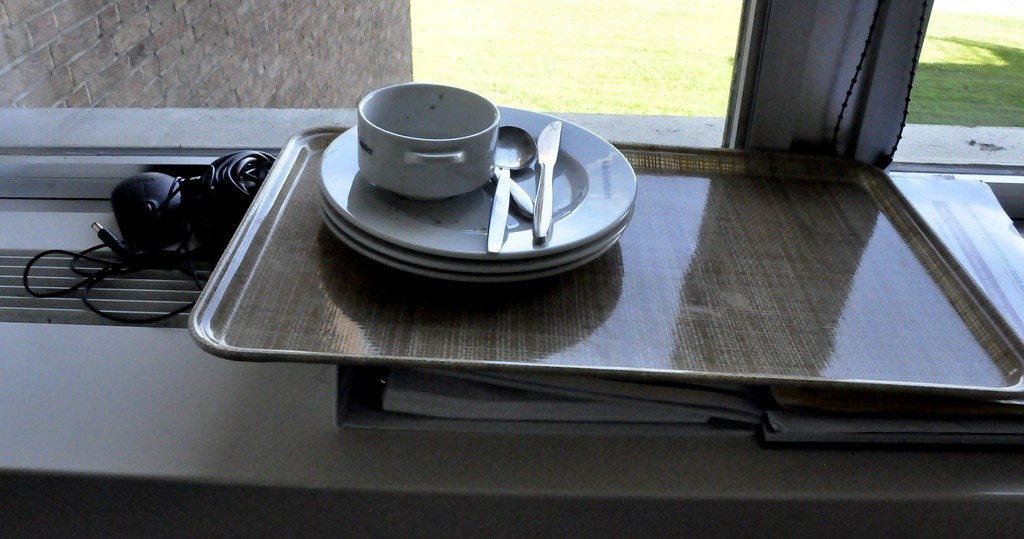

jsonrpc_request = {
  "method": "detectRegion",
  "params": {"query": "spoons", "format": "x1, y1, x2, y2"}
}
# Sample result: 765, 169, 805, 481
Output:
484, 126, 537, 254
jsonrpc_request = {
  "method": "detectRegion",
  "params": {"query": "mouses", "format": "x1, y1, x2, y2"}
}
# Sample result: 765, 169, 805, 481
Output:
111, 172, 187, 251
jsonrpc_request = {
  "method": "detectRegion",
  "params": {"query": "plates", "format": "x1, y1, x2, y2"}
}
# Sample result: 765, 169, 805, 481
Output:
316, 103, 636, 276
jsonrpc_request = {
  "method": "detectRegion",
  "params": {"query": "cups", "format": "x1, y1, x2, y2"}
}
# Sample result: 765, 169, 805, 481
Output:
358, 82, 501, 197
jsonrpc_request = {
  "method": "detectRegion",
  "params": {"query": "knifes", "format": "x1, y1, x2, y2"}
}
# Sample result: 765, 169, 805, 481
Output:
531, 121, 562, 237
